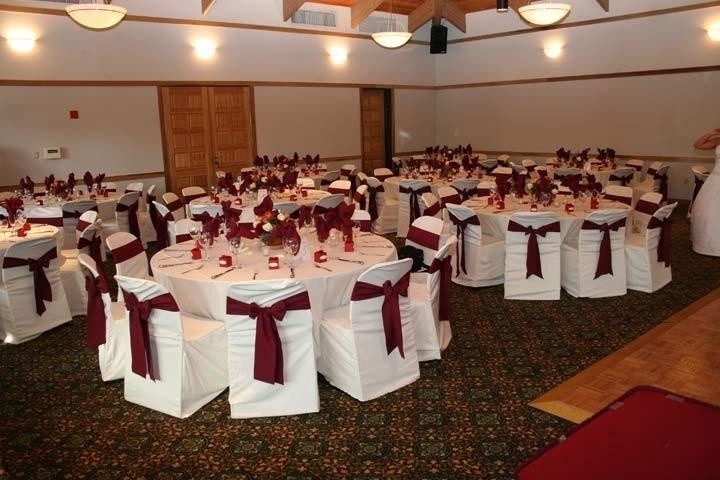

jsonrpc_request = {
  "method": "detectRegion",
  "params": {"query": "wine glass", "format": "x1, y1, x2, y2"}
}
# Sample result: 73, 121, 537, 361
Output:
400, 162, 485, 182
0, 209, 28, 242
17, 182, 108, 206
210, 180, 327, 203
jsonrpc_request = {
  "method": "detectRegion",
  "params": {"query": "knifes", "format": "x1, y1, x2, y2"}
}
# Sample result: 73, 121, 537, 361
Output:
30, 223, 54, 234
151, 220, 393, 280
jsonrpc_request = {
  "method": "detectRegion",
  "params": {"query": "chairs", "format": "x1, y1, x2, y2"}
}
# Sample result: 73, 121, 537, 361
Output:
76, 251, 129, 383
401, 233, 454, 362
1, 234, 75, 345
442, 201, 501, 289
625, 201, 677, 294
1, 148, 717, 272
560, 207, 628, 299
112, 274, 227, 421
311, 256, 423, 406
223, 278, 323, 421
502, 211, 563, 303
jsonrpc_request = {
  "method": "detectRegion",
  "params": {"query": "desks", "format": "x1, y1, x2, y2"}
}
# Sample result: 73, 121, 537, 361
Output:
147, 224, 402, 376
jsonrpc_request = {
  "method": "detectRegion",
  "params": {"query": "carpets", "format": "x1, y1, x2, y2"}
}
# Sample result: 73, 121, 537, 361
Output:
511, 380, 720, 480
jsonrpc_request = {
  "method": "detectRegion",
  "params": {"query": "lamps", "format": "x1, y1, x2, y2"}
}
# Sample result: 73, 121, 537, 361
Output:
705, 25, 720, 41
495, 0, 509, 14
368, 0, 414, 51
64, 0, 128, 31
195, 46, 216, 59
4, 37, 36, 52
517, 1, 572, 29
541, 44, 564, 58
329, 52, 350, 65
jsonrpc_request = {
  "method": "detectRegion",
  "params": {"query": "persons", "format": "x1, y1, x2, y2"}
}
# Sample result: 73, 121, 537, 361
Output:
689, 129, 720, 259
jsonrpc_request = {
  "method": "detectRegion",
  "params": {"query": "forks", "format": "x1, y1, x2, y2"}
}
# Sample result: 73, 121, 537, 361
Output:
469, 190, 625, 218
